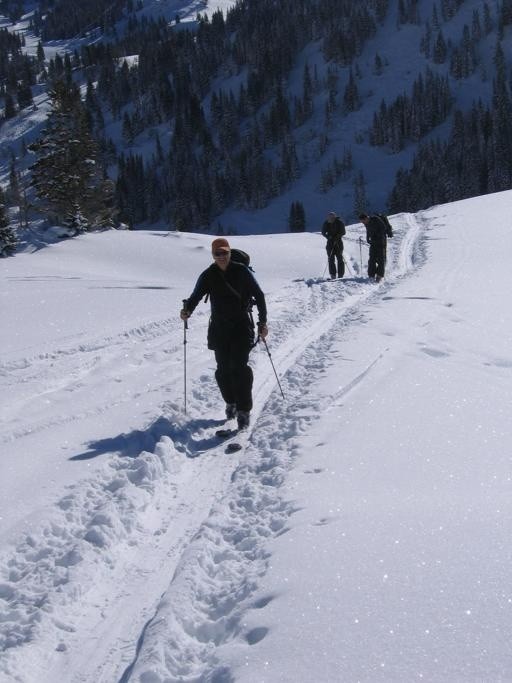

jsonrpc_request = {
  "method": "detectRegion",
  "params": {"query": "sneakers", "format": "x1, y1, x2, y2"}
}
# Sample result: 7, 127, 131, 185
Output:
225, 403, 249, 428
331, 273, 343, 279
369, 274, 384, 282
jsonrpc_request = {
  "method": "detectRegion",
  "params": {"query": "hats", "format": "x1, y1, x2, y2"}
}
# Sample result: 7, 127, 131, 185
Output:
212, 238, 230, 254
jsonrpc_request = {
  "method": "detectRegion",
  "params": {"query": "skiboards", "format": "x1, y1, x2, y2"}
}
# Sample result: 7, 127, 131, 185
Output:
215, 418, 251, 449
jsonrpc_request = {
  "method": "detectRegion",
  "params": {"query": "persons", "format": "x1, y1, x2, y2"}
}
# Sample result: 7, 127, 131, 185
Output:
179, 236, 269, 434
321, 211, 347, 280
359, 213, 388, 284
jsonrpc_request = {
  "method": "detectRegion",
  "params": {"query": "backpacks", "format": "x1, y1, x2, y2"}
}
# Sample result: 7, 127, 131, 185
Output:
375, 214, 393, 235
206, 248, 254, 313
